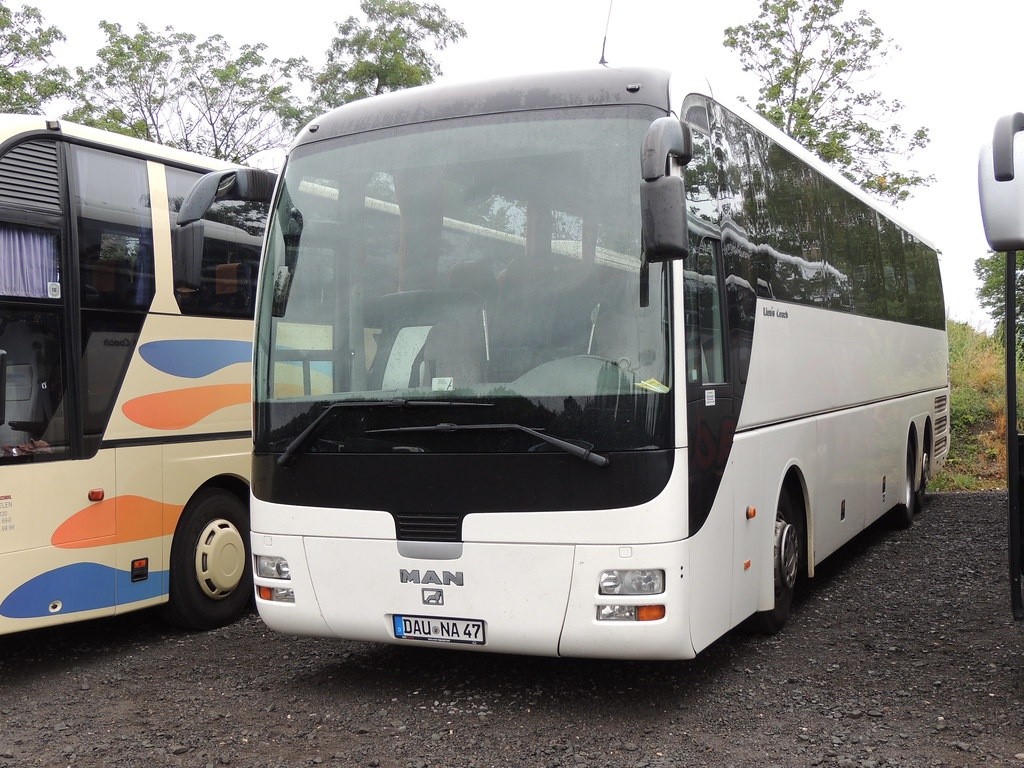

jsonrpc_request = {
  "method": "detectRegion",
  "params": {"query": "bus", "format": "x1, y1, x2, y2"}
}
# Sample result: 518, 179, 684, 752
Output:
175, 57, 957, 667
0, 112, 632, 635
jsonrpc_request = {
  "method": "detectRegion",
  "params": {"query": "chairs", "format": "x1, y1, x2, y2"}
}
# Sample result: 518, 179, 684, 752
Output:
0, 323, 52, 441
404, 254, 718, 379
78, 254, 281, 322
